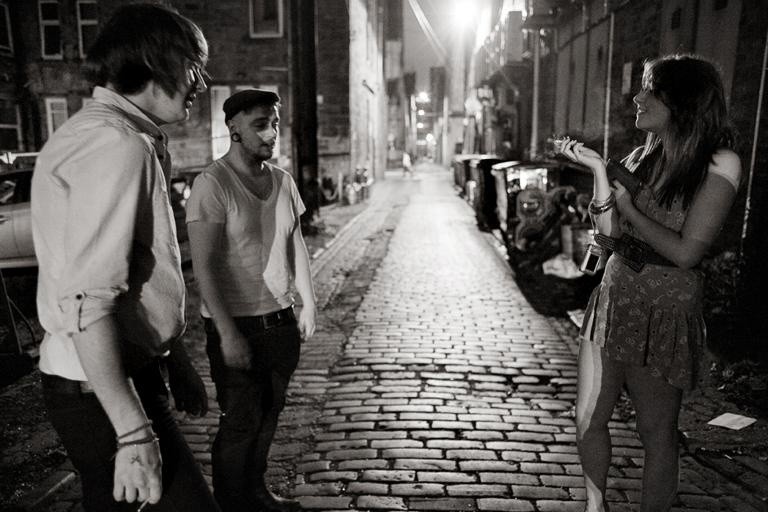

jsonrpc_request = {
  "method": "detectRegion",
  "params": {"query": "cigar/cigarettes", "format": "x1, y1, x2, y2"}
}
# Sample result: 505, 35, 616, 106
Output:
546, 138, 567, 144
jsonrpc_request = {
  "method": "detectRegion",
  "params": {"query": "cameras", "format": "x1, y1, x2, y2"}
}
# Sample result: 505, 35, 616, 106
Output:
579, 244, 602, 276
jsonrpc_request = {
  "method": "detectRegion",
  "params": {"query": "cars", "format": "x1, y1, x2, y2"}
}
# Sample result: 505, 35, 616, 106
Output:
0, 151, 193, 271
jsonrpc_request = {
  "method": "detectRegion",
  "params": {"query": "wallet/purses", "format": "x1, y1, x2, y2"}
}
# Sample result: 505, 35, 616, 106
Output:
606, 157, 642, 200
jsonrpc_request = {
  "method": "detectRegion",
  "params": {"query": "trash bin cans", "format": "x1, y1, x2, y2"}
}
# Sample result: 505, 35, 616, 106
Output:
454, 155, 606, 273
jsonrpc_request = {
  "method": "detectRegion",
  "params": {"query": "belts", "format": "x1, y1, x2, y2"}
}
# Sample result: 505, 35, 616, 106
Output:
204, 305, 295, 331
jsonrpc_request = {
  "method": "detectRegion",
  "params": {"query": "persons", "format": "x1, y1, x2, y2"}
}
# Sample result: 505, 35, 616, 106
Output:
183, 88, 319, 512
548, 50, 743, 511
28, 1, 226, 512
401, 149, 414, 177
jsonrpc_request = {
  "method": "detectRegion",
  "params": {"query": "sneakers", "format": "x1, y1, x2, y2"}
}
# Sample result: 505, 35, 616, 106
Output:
256, 486, 301, 512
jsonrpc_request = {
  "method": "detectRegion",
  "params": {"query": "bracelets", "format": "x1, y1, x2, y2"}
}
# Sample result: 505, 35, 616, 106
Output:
114, 434, 159, 450
588, 199, 616, 214
592, 190, 616, 205
115, 419, 155, 439
